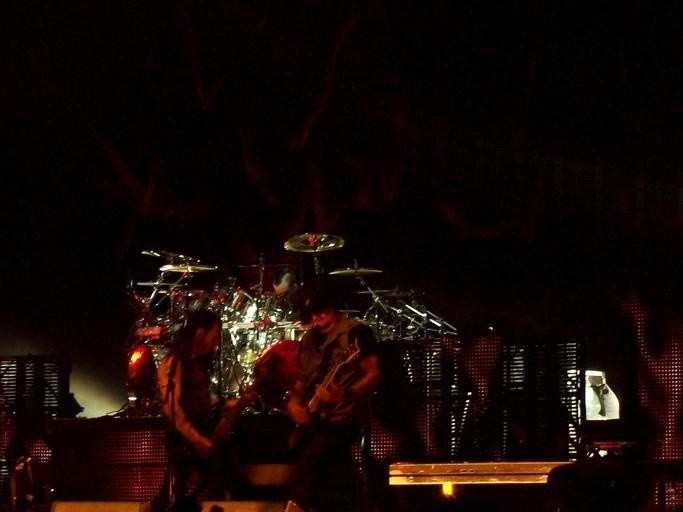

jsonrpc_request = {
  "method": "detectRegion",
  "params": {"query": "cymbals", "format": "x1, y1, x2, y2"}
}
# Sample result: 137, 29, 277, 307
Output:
328, 269, 383, 274
142, 250, 200, 263
237, 261, 286, 268
136, 281, 182, 287
158, 265, 216, 274
283, 233, 344, 253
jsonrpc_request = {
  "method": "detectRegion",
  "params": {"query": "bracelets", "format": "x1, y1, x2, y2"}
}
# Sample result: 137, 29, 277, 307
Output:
343, 385, 353, 402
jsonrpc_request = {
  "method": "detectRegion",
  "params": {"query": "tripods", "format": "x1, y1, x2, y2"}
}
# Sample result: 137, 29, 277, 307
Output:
93, 272, 281, 418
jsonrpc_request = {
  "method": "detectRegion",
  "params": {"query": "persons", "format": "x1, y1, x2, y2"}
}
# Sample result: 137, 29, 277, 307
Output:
140, 309, 240, 509
284, 283, 382, 511
